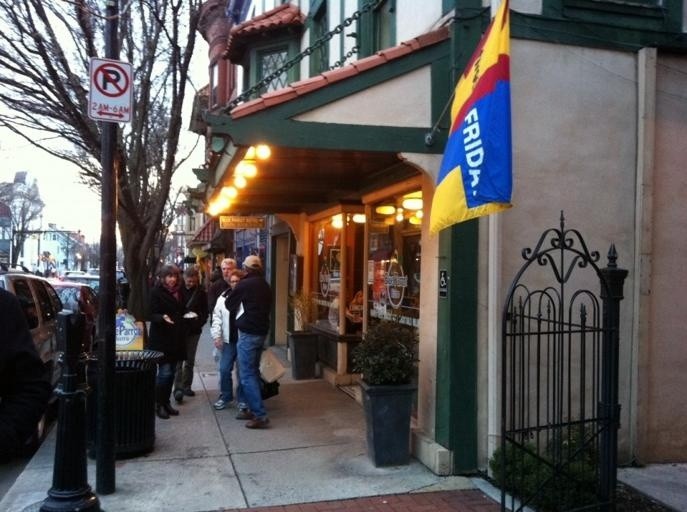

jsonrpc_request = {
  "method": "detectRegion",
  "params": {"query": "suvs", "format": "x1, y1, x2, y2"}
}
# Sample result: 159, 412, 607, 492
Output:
0, 259, 63, 449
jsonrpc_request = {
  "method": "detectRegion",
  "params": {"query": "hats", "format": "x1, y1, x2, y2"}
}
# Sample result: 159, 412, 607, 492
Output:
240, 255, 263, 269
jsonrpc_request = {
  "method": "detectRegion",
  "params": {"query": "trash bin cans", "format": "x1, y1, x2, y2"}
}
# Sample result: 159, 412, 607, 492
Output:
85, 350, 164, 460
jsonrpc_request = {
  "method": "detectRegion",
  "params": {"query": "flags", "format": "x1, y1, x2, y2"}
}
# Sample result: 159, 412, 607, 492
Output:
429, 0, 513, 235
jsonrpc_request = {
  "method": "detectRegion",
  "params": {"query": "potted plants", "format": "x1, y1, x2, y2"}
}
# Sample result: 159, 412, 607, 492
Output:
285, 284, 320, 380
350, 313, 416, 467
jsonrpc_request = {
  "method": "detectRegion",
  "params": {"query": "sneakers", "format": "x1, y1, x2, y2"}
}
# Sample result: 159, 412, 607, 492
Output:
237, 401, 248, 409
213, 399, 226, 410
173, 389, 183, 402
185, 390, 195, 396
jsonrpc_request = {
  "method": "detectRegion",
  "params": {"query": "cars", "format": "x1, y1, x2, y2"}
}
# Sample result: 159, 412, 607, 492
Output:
43, 265, 125, 345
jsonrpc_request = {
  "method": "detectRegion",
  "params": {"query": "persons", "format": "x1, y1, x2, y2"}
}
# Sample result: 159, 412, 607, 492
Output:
328, 289, 360, 335
0, 284, 53, 472
348, 290, 363, 306
146, 253, 274, 428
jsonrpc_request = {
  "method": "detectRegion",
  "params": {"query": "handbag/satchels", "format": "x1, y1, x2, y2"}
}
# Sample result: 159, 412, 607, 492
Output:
260, 379, 284, 402
257, 346, 287, 384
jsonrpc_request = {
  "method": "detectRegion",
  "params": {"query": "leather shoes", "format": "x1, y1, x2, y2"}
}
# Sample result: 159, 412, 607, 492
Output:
235, 410, 255, 419
166, 403, 179, 416
244, 416, 270, 429
156, 405, 170, 419
